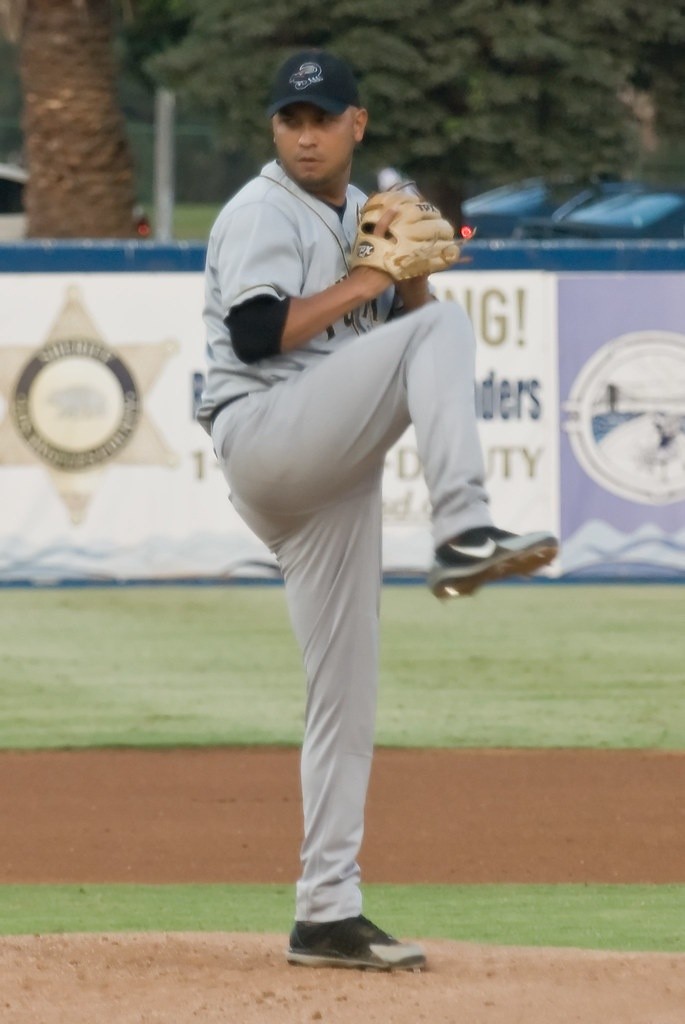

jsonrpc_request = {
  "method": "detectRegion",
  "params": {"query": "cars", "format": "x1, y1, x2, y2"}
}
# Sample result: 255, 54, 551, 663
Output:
0, 166, 150, 244
459, 171, 685, 243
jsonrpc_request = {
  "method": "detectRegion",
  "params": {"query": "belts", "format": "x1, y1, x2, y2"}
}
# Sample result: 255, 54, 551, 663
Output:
208, 393, 247, 422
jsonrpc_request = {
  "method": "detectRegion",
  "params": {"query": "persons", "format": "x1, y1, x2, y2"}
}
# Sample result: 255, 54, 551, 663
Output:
189, 48, 561, 968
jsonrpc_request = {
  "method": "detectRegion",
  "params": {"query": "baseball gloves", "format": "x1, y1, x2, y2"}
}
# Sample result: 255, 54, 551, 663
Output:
350, 192, 461, 282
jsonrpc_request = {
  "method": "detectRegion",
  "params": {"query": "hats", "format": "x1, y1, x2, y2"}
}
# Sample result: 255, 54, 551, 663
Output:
267, 47, 361, 119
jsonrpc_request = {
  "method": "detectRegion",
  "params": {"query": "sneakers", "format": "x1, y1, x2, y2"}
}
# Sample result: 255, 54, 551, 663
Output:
284, 914, 425, 970
427, 527, 557, 599
